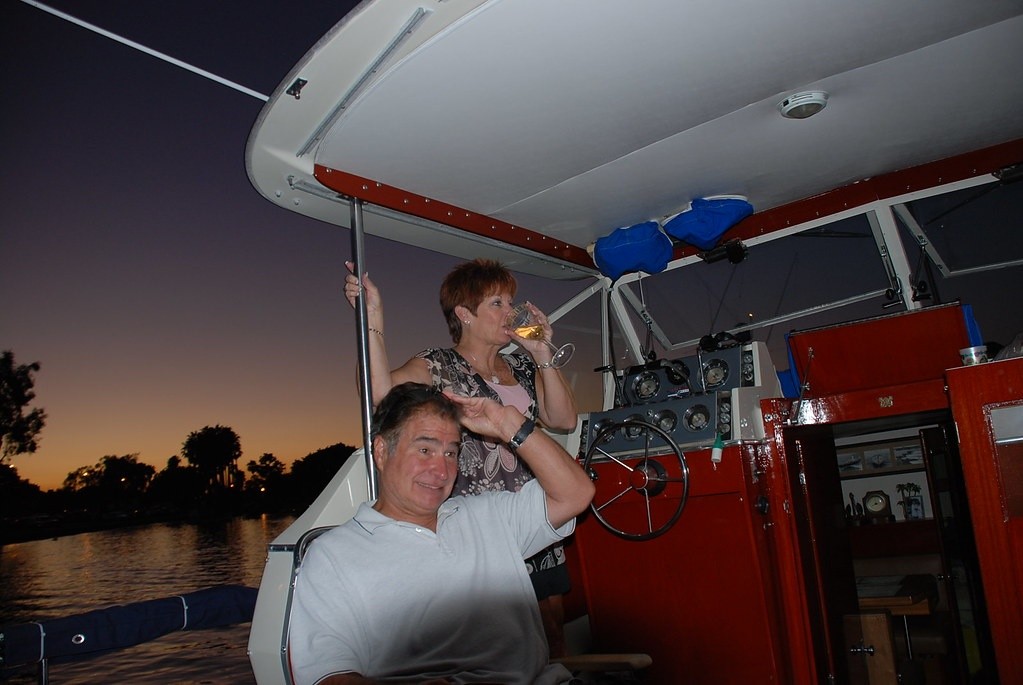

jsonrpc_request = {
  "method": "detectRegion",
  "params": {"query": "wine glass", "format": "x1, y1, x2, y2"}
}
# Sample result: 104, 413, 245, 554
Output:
504, 302, 575, 369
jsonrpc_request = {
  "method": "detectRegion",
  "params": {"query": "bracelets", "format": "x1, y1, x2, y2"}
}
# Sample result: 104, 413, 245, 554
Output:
369, 328, 384, 336
538, 363, 553, 369
508, 417, 535, 451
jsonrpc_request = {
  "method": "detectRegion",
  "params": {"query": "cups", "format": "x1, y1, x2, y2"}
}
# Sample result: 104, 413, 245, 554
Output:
959, 345, 988, 365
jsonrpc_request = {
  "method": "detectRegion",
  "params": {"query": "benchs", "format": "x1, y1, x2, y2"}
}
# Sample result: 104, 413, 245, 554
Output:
853, 570, 938, 667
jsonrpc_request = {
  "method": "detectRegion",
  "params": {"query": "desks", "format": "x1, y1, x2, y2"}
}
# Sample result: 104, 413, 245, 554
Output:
850, 588, 930, 609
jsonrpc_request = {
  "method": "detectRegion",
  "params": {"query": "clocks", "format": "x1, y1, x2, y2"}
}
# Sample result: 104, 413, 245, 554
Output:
861, 488, 893, 522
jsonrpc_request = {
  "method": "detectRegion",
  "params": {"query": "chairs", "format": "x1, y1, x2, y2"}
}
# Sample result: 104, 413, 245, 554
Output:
279, 521, 656, 685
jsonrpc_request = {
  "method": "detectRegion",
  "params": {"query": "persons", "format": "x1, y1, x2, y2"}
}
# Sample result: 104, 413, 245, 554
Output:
344, 259, 578, 606
290, 382, 595, 685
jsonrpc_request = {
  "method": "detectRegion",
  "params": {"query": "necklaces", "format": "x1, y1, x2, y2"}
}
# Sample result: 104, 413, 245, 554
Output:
490, 374, 500, 383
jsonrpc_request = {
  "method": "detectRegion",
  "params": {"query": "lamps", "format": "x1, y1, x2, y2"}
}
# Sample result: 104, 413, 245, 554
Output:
778, 96, 827, 123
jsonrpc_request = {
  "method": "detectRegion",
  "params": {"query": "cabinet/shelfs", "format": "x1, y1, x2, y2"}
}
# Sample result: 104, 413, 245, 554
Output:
804, 417, 1002, 685
